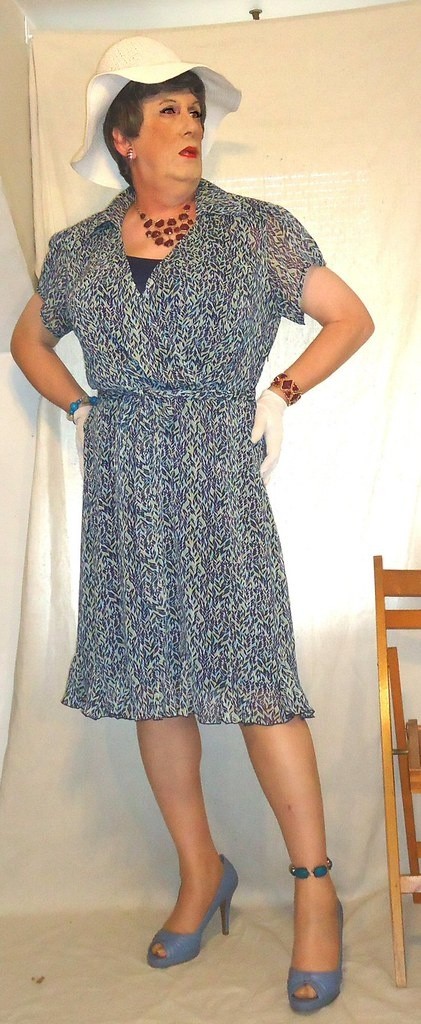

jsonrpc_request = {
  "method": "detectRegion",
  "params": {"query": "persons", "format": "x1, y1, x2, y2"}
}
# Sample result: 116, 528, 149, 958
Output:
9, 34, 376, 1017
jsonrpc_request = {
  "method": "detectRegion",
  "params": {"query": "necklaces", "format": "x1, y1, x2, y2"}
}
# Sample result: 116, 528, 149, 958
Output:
134, 201, 196, 249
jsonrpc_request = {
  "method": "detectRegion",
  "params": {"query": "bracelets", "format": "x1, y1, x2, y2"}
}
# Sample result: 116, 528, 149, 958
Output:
271, 373, 302, 406
66, 396, 98, 426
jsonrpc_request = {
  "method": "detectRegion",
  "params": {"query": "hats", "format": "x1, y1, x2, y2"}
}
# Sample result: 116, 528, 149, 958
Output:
67, 35, 265, 192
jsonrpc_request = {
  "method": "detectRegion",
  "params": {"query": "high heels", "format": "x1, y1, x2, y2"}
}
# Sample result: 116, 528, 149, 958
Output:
286, 899, 353, 1013
146, 855, 239, 968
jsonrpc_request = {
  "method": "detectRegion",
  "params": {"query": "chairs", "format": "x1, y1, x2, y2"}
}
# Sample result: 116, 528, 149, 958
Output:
374, 555, 421, 989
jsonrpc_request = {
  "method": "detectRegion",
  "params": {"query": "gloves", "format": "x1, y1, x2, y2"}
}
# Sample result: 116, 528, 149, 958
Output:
248, 390, 314, 483
72, 407, 94, 493
